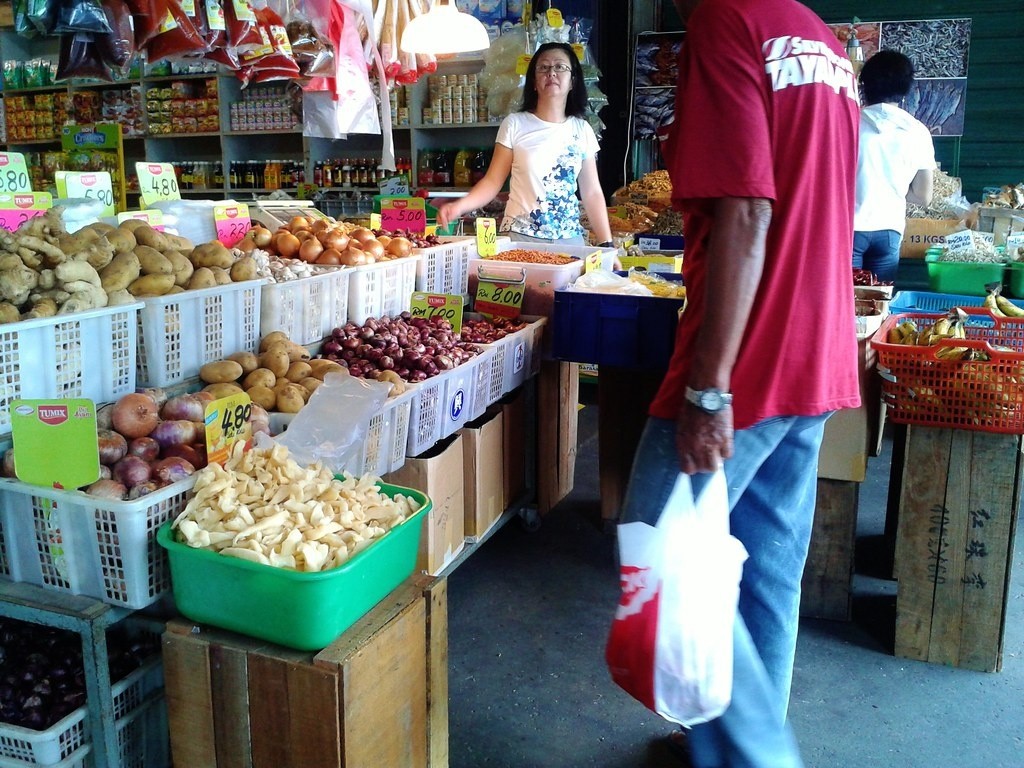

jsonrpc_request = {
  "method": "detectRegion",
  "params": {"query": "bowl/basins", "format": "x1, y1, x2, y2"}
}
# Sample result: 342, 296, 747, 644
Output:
1007, 260, 1024, 299
925, 260, 1008, 296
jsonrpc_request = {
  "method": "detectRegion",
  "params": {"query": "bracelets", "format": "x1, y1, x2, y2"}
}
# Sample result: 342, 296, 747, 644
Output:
597, 241, 614, 248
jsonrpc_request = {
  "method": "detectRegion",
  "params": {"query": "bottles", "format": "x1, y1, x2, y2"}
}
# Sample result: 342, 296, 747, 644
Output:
229, 158, 305, 189
168, 160, 224, 190
314, 154, 413, 188
416, 143, 492, 188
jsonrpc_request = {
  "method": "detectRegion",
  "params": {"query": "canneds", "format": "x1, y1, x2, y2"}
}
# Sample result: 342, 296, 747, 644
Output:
388, 84, 411, 127
423, 72, 505, 125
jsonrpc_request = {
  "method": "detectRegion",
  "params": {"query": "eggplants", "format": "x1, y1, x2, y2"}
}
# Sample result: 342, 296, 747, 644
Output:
0, 619, 164, 768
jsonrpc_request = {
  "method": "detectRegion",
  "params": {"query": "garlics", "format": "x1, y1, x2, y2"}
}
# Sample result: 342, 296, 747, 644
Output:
223, 250, 348, 338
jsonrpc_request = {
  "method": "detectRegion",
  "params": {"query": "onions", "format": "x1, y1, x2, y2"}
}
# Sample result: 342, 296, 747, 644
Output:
0, 388, 271, 598
327, 312, 538, 439
237, 209, 446, 267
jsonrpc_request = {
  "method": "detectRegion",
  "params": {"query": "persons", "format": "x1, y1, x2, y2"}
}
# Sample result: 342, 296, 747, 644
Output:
437, 42, 622, 275
624, 0, 861, 768
853, 50, 936, 286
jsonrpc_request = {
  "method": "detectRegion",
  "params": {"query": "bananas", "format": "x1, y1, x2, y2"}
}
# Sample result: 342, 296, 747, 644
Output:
884, 284, 1024, 429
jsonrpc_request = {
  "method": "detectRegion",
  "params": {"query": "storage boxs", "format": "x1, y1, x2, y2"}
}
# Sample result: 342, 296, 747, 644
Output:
0, 192, 1024, 768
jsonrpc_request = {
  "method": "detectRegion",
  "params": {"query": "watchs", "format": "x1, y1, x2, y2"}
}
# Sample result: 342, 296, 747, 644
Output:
684, 386, 733, 414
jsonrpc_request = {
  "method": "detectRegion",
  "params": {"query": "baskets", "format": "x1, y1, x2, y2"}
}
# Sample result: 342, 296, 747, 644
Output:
870, 307, 1024, 435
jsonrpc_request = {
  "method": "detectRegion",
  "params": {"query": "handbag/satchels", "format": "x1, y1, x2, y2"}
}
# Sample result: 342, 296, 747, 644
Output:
605, 461, 749, 728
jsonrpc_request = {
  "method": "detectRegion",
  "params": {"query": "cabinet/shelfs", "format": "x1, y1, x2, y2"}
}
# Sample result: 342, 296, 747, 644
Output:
0, 49, 500, 198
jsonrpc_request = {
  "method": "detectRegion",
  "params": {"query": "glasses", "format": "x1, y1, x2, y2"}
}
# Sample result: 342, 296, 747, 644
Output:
534, 64, 572, 73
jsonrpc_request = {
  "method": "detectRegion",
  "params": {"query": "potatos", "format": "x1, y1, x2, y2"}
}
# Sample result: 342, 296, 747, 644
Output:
63, 219, 256, 375
199, 332, 406, 477
